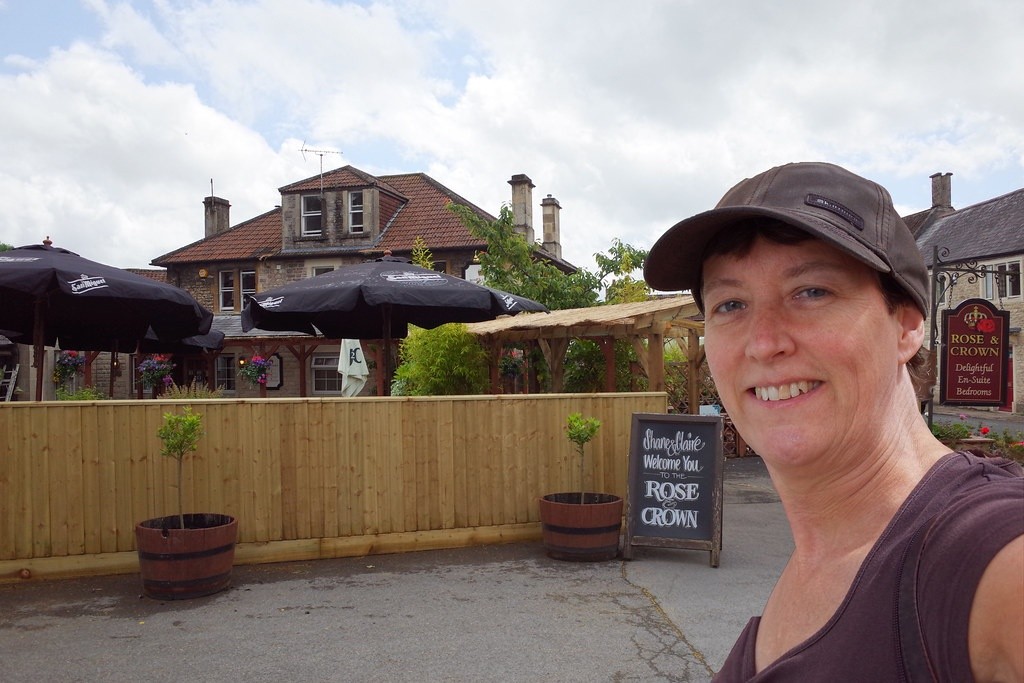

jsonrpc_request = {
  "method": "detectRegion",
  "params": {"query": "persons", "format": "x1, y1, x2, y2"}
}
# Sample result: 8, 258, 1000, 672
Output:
642, 161, 1023, 682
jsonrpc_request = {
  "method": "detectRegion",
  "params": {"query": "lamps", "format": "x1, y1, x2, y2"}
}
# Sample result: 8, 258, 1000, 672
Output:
114, 359, 120, 369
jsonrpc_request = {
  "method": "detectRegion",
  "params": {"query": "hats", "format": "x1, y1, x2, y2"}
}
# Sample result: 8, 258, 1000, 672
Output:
642, 160, 928, 321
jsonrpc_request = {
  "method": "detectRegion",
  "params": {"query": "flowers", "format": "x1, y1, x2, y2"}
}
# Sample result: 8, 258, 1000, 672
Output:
138, 353, 177, 388
502, 351, 517, 358
52, 350, 86, 383
241, 355, 272, 383
959, 413, 990, 437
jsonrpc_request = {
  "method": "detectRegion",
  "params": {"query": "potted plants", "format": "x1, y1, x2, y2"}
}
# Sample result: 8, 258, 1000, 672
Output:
538, 413, 624, 562
134, 404, 239, 602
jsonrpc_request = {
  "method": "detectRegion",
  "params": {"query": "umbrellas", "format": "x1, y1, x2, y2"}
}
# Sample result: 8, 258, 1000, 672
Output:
0, 237, 213, 399
240, 248, 550, 396
103, 324, 228, 401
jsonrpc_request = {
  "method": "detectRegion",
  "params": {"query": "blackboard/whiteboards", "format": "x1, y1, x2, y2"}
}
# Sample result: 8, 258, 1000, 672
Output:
627, 412, 724, 551
